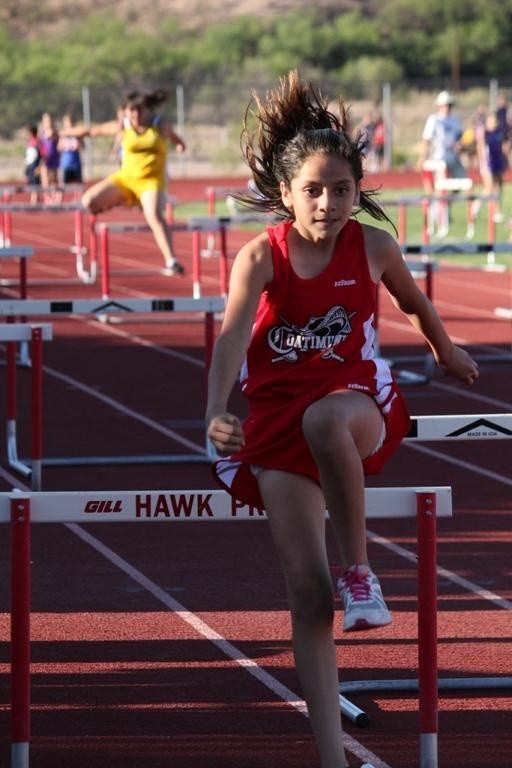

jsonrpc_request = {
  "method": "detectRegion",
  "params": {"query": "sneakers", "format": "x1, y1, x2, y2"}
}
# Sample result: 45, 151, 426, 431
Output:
337, 564, 392, 633
163, 262, 182, 276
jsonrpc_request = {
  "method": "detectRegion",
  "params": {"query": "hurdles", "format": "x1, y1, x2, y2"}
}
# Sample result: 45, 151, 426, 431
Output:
0, 295, 231, 464
0, 487, 452, 768
362, 197, 512, 363
1, 321, 55, 491
1, 186, 292, 300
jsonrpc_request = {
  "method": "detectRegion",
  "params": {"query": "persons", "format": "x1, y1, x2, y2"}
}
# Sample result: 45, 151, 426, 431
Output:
204, 68, 480, 767
23, 89, 185, 276
414, 89, 478, 238
353, 110, 387, 160
475, 89, 510, 226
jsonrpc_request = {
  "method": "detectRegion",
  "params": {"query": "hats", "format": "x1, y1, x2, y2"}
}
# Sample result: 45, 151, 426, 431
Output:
434, 91, 455, 106
